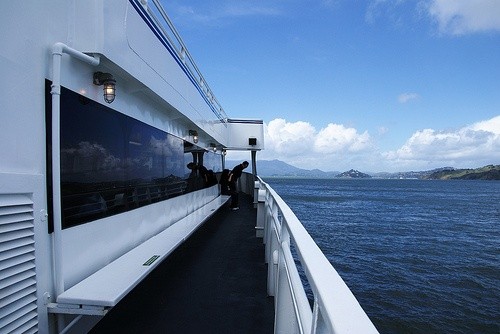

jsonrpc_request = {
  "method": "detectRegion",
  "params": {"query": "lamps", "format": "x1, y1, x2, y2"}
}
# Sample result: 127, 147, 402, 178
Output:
92, 71, 118, 104
188, 130, 227, 157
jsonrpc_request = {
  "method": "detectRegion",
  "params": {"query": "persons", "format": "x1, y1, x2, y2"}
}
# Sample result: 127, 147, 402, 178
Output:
228, 160, 250, 191
220, 169, 240, 211
185, 162, 217, 193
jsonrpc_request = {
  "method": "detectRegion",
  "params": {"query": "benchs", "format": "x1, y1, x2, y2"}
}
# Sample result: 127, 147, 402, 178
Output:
55, 190, 234, 316
